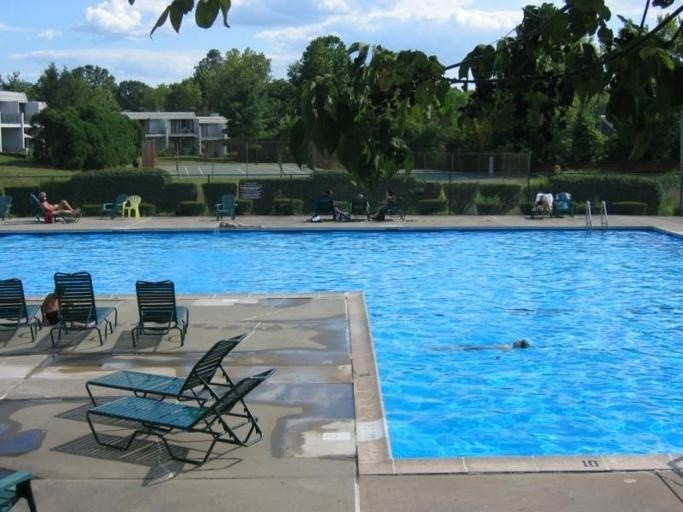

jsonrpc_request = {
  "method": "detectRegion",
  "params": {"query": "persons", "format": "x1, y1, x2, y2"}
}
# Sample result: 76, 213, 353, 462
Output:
326, 187, 394, 219
430, 338, 529, 360
39, 192, 80, 216
537, 196, 548, 215
43, 283, 75, 324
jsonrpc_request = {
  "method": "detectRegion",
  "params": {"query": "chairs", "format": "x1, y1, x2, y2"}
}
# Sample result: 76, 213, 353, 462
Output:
122, 195, 142, 220
531, 193, 554, 218
311, 196, 337, 221
348, 199, 371, 220
101, 194, 128, 220
84, 333, 264, 448
0, 195, 13, 221
131, 280, 188, 346
0, 465, 37, 511
30, 193, 82, 223
49, 271, 117, 346
216, 194, 238, 220
84, 367, 277, 464
386, 197, 410, 221
0, 278, 42, 340
554, 193, 576, 218
